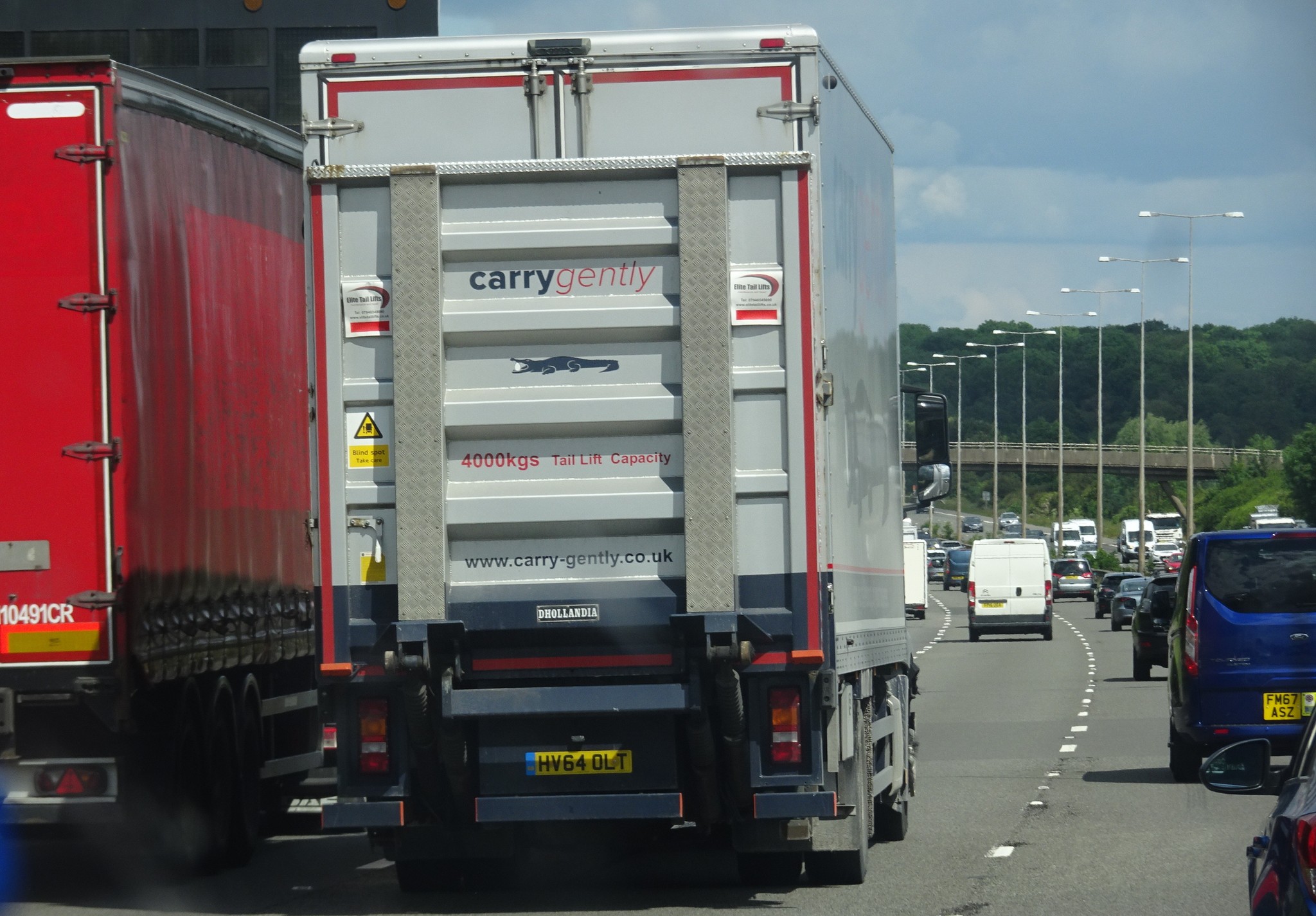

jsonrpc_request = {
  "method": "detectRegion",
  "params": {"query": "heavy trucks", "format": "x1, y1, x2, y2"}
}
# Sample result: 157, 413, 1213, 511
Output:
1, 48, 388, 912
287, 18, 952, 900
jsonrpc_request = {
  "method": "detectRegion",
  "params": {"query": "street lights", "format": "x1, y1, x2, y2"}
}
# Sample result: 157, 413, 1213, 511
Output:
966, 342, 1024, 538
1099, 254, 1190, 575
906, 361, 956, 392
993, 329, 1056, 539
1025, 310, 1098, 561
933, 352, 987, 546
1137, 208, 1245, 541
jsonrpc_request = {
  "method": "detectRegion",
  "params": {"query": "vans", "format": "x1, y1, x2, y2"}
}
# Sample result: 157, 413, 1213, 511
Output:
960, 538, 1054, 643
1049, 520, 1084, 557
1118, 518, 1157, 564
1068, 518, 1097, 545
1149, 525, 1316, 785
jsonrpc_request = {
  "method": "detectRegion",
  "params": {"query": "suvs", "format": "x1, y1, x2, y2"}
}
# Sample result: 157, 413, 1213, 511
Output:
1091, 571, 1144, 619
941, 548, 972, 593
1103, 576, 1151, 631
1123, 571, 1182, 681
1051, 558, 1095, 603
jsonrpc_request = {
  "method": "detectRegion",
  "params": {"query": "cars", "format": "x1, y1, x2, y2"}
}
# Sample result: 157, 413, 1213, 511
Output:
998, 511, 1020, 529
1198, 701, 1316, 916
1074, 543, 1097, 561
961, 515, 984, 533
902, 494, 1310, 631
